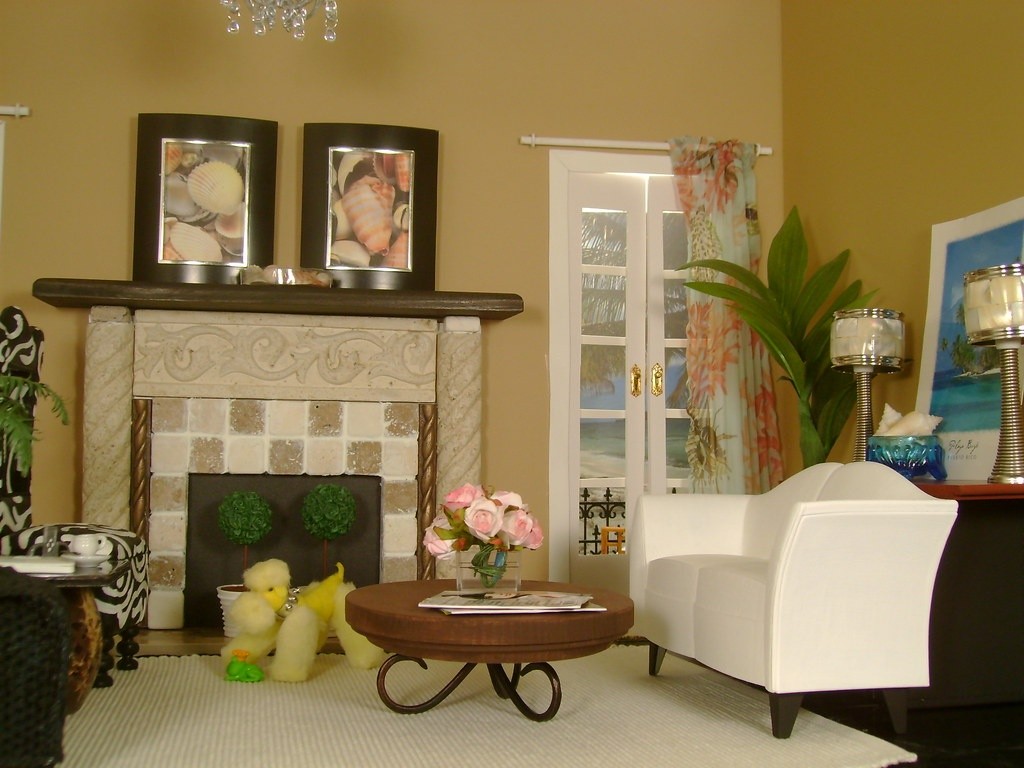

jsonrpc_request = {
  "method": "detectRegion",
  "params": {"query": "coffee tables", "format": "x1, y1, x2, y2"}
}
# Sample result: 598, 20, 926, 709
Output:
23, 554, 133, 714
345, 579, 635, 722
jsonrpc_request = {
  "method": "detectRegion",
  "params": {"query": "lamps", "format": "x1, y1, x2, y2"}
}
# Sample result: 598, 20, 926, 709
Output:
830, 308, 905, 462
218, 0, 340, 43
963, 263, 1024, 484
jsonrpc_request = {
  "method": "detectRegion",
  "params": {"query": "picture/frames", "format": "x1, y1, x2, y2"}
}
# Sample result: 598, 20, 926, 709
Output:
132, 113, 278, 284
299, 122, 439, 292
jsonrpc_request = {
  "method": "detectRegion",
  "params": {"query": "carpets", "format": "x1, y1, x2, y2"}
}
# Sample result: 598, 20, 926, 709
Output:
55, 645, 917, 768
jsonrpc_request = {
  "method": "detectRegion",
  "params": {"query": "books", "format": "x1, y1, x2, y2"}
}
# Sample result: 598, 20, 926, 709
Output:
416, 589, 608, 616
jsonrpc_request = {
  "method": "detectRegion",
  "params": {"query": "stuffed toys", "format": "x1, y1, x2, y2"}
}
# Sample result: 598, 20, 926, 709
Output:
222, 559, 385, 682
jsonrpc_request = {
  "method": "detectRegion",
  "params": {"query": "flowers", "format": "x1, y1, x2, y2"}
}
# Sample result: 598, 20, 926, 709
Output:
423, 483, 544, 561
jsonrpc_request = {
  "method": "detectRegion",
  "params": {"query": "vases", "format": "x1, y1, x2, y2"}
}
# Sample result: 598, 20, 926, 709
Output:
456, 544, 521, 599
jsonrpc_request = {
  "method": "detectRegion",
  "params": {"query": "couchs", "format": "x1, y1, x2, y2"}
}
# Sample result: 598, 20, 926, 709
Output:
628, 461, 959, 740
0, 306, 151, 689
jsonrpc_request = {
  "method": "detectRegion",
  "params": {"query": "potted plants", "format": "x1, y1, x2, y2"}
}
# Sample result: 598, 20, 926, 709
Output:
216, 490, 272, 638
295, 483, 361, 639
